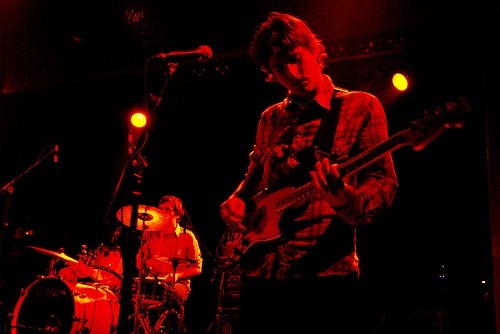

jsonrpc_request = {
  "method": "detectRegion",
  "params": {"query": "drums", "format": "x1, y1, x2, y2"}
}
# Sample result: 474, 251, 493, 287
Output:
131, 277, 167, 305
89, 244, 123, 287
7, 273, 120, 334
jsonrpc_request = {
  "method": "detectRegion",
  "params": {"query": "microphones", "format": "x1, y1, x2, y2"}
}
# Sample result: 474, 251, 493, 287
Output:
53, 144, 59, 164
150, 45, 213, 63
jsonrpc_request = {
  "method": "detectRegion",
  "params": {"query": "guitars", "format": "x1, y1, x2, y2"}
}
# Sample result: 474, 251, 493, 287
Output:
216, 96, 472, 275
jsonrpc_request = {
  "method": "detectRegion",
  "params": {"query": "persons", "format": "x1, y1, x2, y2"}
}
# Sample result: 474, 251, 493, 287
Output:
135, 195, 202, 304
220, 12, 398, 334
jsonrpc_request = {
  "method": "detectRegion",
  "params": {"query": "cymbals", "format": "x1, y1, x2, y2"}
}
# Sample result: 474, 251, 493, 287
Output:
24, 246, 80, 263
115, 204, 173, 231
155, 256, 200, 268
